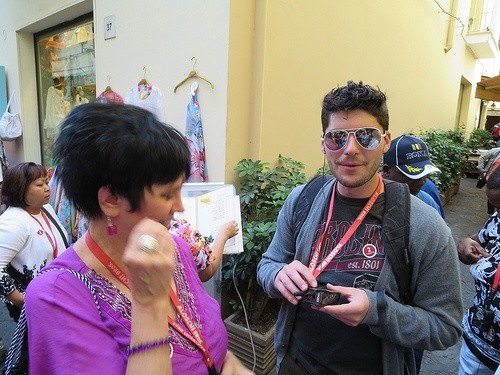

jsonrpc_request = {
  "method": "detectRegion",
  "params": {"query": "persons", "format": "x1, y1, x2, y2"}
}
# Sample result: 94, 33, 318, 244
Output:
257, 81, 465, 375
467, 18, 473, 33
455, 163, 500, 375
376, 132, 446, 224
21, 100, 257, 375
165, 213, 240, 283
477, 123, 500, 217
0, 162, 70, 375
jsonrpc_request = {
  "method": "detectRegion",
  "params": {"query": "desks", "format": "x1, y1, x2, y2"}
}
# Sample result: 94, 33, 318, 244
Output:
468, 156, 488, 163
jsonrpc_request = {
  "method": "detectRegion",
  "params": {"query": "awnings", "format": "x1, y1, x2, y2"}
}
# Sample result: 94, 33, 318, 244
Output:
474, 74, 500, 102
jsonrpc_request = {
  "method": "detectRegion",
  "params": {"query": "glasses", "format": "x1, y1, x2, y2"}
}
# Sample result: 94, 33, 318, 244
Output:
321, 126, 387, 152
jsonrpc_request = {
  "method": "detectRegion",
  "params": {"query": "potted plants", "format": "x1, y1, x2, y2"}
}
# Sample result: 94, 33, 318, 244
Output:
472, 127, 492, 149
421, 123, 469, 206
465, 162, 480, 178
224, 153, 307, 375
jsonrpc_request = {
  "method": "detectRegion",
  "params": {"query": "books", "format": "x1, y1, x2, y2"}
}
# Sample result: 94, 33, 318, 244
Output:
174, 185, 244, 254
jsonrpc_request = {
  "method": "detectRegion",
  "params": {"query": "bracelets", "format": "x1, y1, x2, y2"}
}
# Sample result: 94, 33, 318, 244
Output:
125, 335, 180, 355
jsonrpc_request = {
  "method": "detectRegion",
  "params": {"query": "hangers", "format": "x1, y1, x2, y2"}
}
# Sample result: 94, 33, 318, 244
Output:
105, 74, 113, 92
140, 66, 148, 85
52, 82, 66, 90
173, 56, 214, 93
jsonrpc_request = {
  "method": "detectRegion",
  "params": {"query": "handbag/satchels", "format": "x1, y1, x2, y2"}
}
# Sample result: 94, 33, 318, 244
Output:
0, 93, 22, 142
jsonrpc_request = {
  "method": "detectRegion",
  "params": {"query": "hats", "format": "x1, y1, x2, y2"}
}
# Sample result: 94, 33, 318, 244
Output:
383, 133, 442, 180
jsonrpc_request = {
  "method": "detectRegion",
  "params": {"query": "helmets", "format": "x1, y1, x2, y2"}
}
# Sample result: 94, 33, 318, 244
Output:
490, 123, 500, 142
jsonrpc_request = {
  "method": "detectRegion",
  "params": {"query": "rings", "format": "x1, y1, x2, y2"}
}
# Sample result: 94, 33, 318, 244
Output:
138, 234, 160, 254
